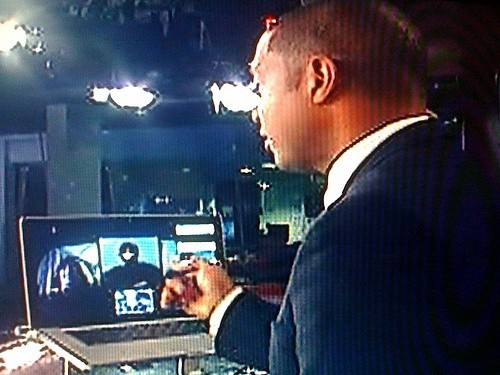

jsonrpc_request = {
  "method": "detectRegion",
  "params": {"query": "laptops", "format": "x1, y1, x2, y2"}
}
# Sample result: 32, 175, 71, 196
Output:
18, 214, 226, 371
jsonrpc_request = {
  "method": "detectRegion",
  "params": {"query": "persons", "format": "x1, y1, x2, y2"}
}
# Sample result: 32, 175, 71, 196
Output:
158, 0, 500, 374
